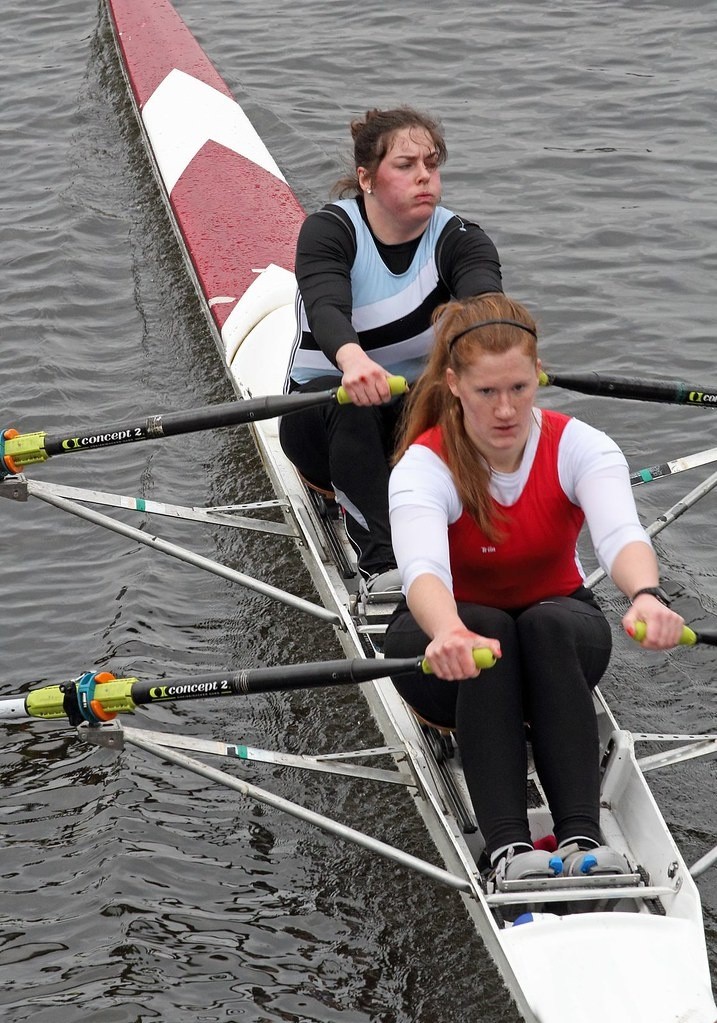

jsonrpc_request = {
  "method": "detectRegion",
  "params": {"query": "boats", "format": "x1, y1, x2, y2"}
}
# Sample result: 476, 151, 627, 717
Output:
102, 0, 717, 1022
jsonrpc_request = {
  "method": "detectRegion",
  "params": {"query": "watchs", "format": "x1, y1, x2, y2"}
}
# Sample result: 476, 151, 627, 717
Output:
630, 587, 672, 609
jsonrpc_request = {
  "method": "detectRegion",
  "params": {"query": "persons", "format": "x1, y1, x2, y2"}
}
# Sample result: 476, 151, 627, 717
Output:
382, 294, 685, 882
277, 108, 506, 593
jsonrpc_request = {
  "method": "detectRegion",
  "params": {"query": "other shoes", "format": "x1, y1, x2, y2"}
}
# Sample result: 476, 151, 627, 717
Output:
370, 568, 403, 591
561, 846, 626, 913
498, 849, 562, 923
